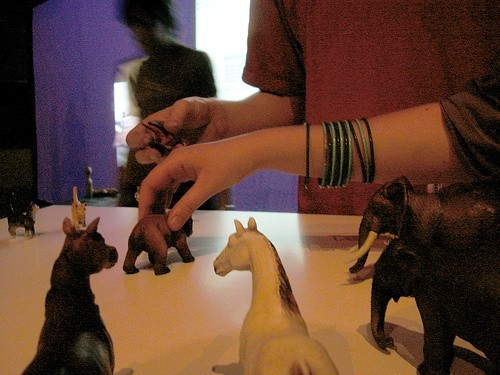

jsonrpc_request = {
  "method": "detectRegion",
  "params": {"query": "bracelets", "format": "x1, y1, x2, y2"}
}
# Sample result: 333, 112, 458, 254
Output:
303, 116, 376, 190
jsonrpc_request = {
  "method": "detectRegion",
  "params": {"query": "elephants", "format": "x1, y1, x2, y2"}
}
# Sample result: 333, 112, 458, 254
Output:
343, 175, 500, 375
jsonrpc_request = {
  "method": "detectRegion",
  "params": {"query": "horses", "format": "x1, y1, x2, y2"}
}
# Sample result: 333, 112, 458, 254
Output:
213, 218, 341, 375
21, 217, 119, 375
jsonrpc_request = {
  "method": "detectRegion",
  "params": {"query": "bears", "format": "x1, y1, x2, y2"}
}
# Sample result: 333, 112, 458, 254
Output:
123, 211, 194, 276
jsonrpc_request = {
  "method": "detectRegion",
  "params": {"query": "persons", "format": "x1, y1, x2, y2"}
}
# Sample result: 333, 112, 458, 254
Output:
127, 0, 500, 216
136, 69, 500, 231
116, 0, 217, 211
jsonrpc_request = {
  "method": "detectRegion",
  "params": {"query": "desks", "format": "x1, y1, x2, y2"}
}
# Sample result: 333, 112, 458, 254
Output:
0, 205, 495, 375
